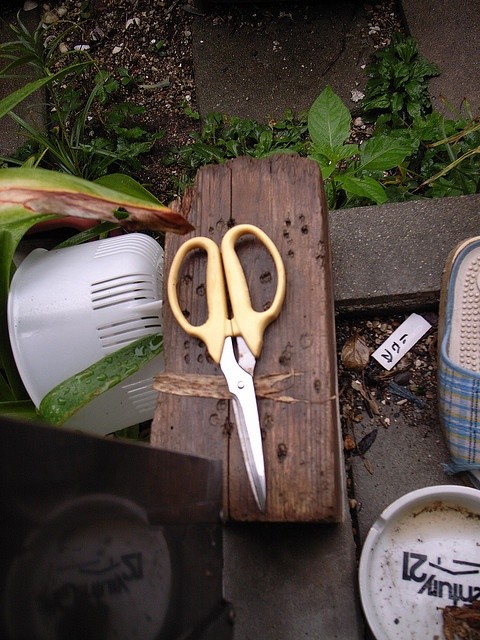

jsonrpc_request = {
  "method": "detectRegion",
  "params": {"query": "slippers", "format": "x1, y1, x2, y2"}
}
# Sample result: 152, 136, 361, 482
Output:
437, 234, 480, 489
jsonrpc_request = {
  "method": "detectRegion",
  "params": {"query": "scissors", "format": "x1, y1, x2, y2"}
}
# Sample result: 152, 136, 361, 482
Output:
166, 223, 286, 514
